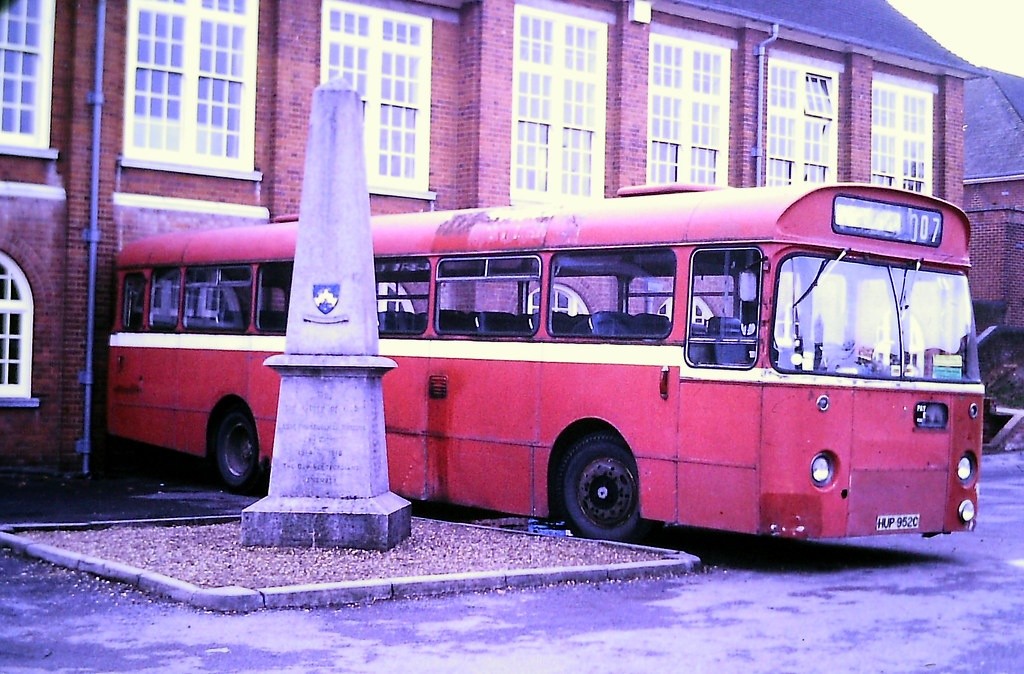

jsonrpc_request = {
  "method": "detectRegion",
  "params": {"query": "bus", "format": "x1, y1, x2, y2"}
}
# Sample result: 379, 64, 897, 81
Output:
105, 179, 989, 549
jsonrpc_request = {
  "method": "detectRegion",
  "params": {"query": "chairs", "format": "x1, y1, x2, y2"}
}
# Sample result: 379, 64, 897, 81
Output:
128, 303, 750, 370
